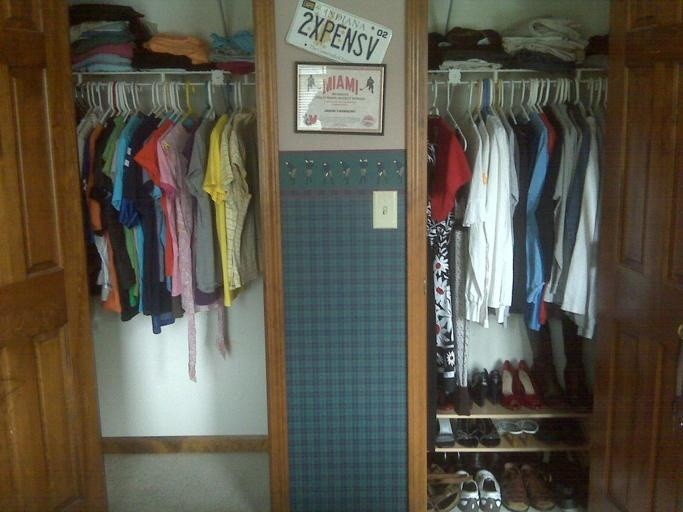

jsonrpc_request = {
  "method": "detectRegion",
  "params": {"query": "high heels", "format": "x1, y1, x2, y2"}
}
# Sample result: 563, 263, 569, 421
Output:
435, 418, 455, 448
564, 364, 592, 412
502, 360, 522, 411
469, 369, 488, 406
437, 371, 458, 407
456, 385, 472, 414
532, 359, 569, 409
490, 369, 502, 404
517, 360, 544, 410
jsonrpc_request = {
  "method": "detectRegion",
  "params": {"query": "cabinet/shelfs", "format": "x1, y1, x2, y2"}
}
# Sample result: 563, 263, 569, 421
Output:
405, 1, 683, 512
0, 0, 290, 512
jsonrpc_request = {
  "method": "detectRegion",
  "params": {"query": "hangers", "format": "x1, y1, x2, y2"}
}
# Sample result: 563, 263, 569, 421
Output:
426, 76, 609, 153
73, 80, 257, 145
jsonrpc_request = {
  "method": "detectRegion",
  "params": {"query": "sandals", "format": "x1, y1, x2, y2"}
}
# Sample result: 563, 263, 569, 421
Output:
457, 418, 478, 449
523, 463, 556, 510
556, 498, 578, 509
477, 418, 501, 447
428, 463, 472, 511
549, 479, 575, 496
499, 463, 531, 512
427, 490, 438, 512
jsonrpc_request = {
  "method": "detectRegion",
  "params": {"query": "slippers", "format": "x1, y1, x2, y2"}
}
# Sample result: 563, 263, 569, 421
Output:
498, 420, 522, 447
515, 420, 539, 446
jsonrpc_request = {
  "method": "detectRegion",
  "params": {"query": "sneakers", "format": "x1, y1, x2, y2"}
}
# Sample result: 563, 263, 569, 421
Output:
456, 470, 480, 512
477, 467, 502, 512
